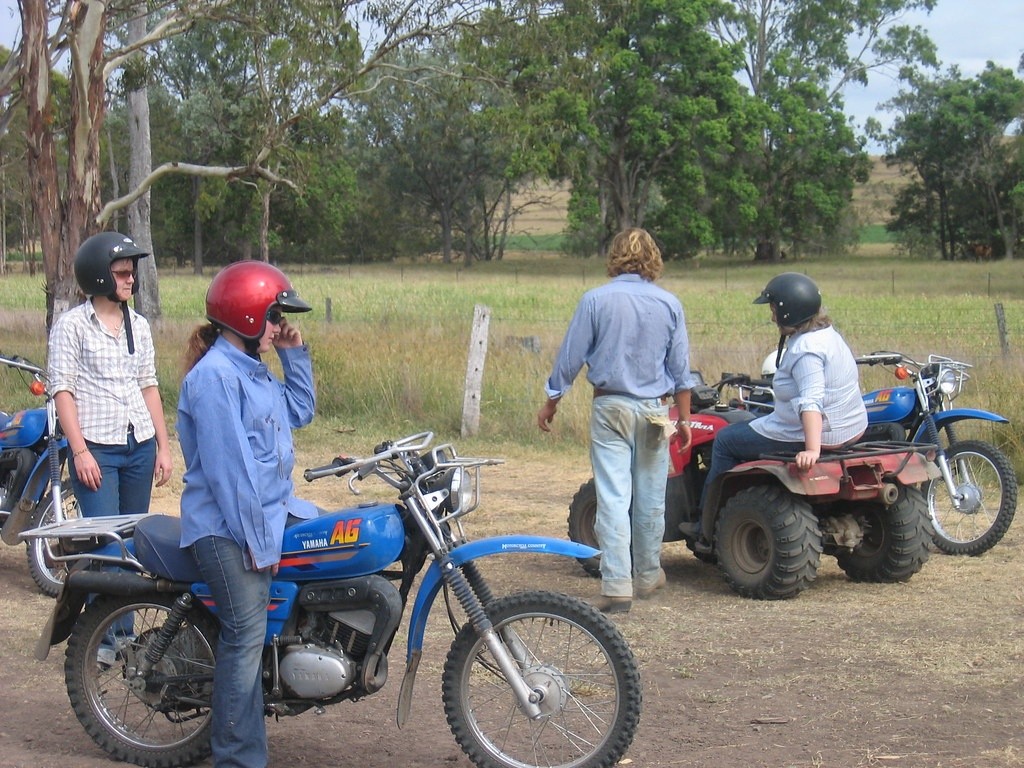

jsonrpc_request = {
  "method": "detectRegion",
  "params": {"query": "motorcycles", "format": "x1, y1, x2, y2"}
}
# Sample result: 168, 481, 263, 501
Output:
712, 348, 1019, 562
1, 351, 110, 603
34, 428, 644, 767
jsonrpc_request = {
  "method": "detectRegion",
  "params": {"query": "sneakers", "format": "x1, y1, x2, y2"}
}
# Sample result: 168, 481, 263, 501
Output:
635, 565, 667, 599
588, 593, 633, 614
678, 520, 715, 546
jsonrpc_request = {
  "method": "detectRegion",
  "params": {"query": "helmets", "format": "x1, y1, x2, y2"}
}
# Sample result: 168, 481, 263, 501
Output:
74, 232, 151, 296
752, 272, 822, 327
205, 260, 312, 341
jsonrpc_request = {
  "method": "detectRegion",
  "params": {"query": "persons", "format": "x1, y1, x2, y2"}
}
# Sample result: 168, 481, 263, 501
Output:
178, 260, 338, 768
534, 229, 694, 612
47, 232, 174, 681
693, 275, 868, 561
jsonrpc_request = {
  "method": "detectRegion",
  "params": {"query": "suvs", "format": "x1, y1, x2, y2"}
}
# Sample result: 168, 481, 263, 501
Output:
568, 369, 943, 603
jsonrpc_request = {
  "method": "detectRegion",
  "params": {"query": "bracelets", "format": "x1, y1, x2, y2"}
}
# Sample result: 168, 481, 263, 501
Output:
72, 448, 89, 456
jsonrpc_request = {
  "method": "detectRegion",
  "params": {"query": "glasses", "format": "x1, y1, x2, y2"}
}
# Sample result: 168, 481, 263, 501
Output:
112, 268, 138, 280
267, 310, 282, 326
